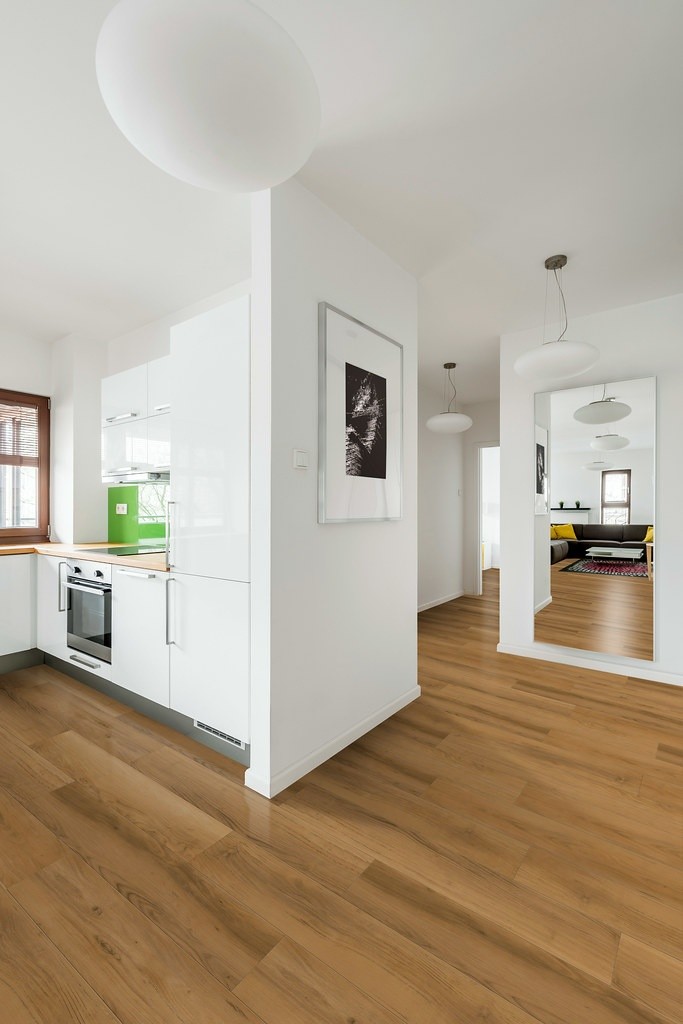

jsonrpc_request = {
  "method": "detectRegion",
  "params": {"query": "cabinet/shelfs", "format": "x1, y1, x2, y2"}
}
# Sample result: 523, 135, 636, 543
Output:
36, 554, 67, 663
109, 566, 248, 743
166, 293, 252, 583
98, 358, 168, 483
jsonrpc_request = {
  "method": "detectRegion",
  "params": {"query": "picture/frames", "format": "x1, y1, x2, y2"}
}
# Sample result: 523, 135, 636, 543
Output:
535, 426, 548, 515
310, 300, 403, 525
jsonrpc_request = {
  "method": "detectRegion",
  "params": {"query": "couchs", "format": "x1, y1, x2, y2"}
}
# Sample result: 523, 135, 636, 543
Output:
550, 523, 654, 564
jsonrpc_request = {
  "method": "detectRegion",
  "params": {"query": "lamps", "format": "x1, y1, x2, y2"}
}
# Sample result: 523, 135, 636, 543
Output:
95, 0, 323, 192
588, 459, 615, 471
426, 362, 473, 432
571, 390, 637, 423
511, 255, 598, 385
591, 431, 630, 449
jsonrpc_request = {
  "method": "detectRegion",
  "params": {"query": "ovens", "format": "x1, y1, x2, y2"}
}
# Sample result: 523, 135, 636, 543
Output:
66, 558, 112, 664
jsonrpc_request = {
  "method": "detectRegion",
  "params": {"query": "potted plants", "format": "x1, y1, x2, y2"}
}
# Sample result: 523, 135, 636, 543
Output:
575, 500, 581, 507
559, 501, 564, 508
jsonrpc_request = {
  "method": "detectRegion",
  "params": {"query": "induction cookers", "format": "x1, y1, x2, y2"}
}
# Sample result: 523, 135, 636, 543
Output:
77, 545, 172, 555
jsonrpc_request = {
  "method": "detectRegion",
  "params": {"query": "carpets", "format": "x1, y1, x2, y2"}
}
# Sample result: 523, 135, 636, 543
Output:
559, 557, 652, 577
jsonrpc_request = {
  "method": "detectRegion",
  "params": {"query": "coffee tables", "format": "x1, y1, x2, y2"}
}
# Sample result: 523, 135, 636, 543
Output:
587, 548, 643, 567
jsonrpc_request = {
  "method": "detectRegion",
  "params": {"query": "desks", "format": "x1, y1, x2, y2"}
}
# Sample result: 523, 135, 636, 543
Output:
645, 543, 653, 580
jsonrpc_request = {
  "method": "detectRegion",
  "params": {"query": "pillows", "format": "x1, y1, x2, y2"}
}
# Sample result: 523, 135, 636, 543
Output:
550, 524, 577, 540
643, 527, 653, 544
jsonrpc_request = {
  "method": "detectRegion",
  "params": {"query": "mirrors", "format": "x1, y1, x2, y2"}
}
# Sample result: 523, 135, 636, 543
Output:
533, 376, 657, 663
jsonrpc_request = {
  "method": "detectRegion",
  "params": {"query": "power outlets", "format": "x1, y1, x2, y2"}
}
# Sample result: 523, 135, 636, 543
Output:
115, 503, 128, 515
